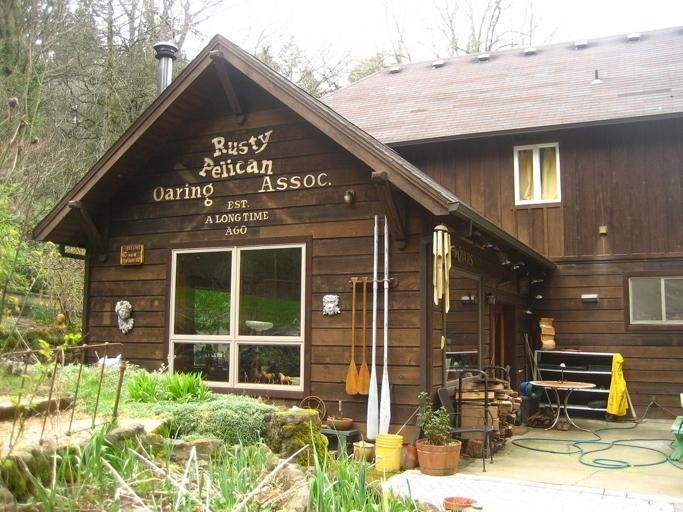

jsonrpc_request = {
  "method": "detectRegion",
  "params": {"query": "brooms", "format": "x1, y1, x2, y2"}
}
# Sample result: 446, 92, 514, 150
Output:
356, 277, 372, 395
344, 276, 359, 395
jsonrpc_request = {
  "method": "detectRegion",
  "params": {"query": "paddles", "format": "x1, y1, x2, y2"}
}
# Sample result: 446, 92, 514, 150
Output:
346, 214, 391, 440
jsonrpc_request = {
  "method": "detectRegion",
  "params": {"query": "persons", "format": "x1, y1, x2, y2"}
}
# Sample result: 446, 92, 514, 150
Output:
322, 294, 341, 316
115, 301, 132, 320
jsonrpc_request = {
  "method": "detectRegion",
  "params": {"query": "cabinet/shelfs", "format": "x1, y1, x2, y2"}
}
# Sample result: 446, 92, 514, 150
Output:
533, 348, 620, 422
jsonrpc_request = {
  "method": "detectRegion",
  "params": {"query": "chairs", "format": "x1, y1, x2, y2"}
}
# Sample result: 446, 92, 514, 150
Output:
437, 388, 496, 473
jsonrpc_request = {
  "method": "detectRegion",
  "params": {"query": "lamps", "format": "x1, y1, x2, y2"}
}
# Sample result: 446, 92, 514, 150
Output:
344, 188, 356, 205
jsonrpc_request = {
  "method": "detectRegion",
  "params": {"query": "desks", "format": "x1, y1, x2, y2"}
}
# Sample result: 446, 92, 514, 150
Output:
529, 380, 597, 432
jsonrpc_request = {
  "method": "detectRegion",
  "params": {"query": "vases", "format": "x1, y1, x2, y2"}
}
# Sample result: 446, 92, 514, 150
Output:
353, 441, 375, 465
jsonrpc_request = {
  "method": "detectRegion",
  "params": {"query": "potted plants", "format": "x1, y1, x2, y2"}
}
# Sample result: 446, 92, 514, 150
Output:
415, 391, 462, 476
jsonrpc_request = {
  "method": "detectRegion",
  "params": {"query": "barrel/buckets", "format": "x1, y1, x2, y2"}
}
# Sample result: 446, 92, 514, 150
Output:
375, 434, 403, 472
